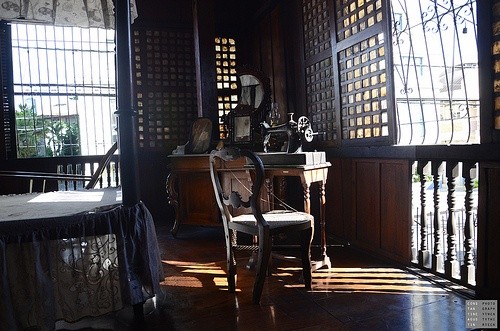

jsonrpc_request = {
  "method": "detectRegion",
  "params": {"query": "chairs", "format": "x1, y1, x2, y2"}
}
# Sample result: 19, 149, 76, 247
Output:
208, 150, 315, 310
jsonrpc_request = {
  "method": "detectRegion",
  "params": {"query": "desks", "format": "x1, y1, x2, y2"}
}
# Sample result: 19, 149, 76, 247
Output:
166, 148, 334, 277
